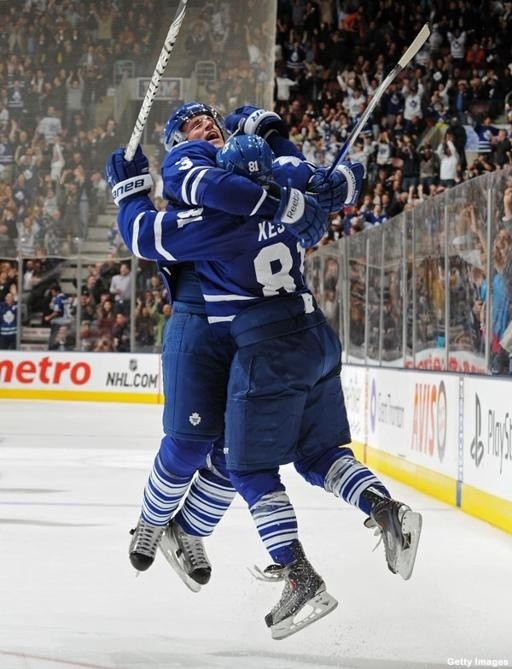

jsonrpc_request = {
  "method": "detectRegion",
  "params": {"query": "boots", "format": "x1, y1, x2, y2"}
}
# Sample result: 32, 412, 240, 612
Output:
363, 483, 412, 574
249, 539, 323, 625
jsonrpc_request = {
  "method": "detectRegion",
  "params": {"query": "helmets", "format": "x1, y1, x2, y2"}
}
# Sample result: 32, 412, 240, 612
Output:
215, 135, 273, 178
163, 104, 225, 151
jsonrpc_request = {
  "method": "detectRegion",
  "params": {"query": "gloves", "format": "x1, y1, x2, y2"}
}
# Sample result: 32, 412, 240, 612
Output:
273, 187, 330, 248
225, 105, 285, 136
307, 163, 364, 214
105, 145, 154, 206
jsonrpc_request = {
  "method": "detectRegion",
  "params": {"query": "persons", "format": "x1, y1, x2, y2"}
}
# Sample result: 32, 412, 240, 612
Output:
106, 141, 411, 625
1, 0, 511, 381
127, 102, 364, 587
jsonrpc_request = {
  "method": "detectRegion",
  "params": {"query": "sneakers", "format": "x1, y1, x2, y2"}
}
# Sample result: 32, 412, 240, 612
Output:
169, 520, 211, 584
129, 511, 167, 571
